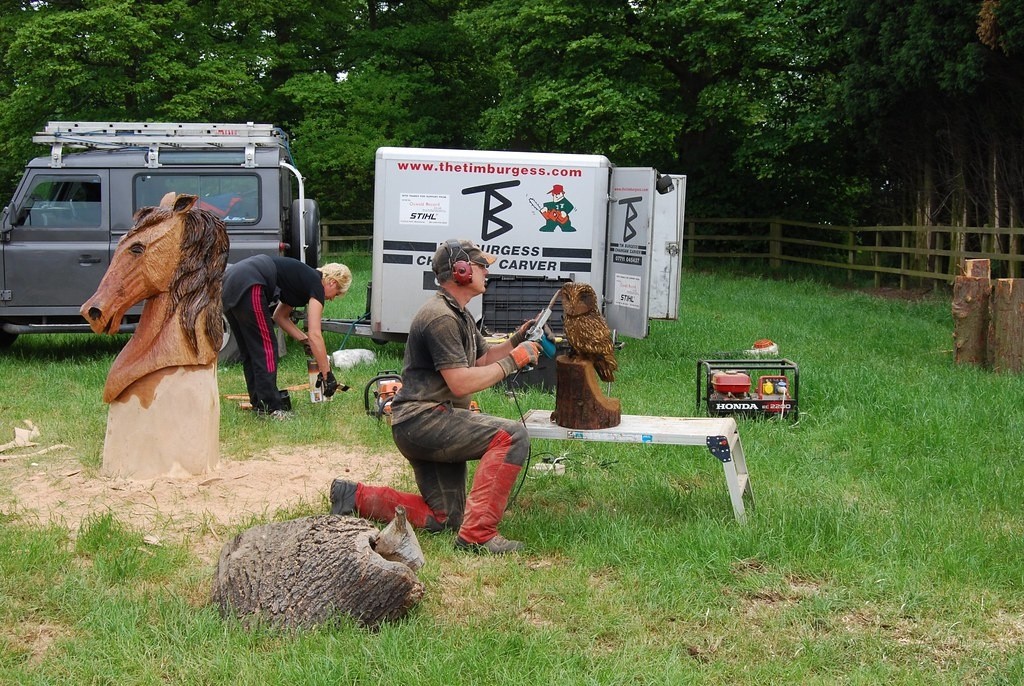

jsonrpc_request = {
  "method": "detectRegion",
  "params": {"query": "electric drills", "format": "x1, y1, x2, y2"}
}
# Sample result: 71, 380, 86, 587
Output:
508, 288, 561, 388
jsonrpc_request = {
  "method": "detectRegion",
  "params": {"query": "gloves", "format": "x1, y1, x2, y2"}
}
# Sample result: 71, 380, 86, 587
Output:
315, 372, 337, 397
509, 320, 555, 349
496, 341, 543, 380
299, 338, 313, 356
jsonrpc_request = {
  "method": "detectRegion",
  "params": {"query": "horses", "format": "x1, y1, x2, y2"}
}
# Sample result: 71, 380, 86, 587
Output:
79, 191, 232, 404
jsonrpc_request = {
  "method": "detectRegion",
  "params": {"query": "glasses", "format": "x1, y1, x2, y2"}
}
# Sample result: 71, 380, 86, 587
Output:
470, 262, 490, 269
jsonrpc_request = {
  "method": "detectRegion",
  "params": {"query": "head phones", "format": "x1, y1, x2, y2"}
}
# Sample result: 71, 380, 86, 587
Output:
447, 239, 474, 286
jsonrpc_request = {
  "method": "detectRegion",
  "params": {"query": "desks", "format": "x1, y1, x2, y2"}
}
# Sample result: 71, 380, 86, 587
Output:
517, 410, 756, 526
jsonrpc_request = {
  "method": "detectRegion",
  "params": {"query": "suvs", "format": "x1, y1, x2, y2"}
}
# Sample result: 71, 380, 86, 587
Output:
0, 121, 321, 371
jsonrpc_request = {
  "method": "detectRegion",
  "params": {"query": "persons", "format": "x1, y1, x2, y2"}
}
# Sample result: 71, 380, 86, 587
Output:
220, 251, 354, 412
329, 237, 543, 554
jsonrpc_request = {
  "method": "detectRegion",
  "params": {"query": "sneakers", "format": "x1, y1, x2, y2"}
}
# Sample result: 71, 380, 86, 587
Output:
329, 480, 358, 515
455, 531, 524, 555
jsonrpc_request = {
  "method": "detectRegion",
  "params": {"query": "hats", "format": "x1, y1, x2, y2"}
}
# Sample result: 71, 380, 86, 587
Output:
432, 239, 497, 280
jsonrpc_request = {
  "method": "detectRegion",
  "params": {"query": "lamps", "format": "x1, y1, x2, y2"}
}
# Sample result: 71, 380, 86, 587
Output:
655, 172, 675, 195
